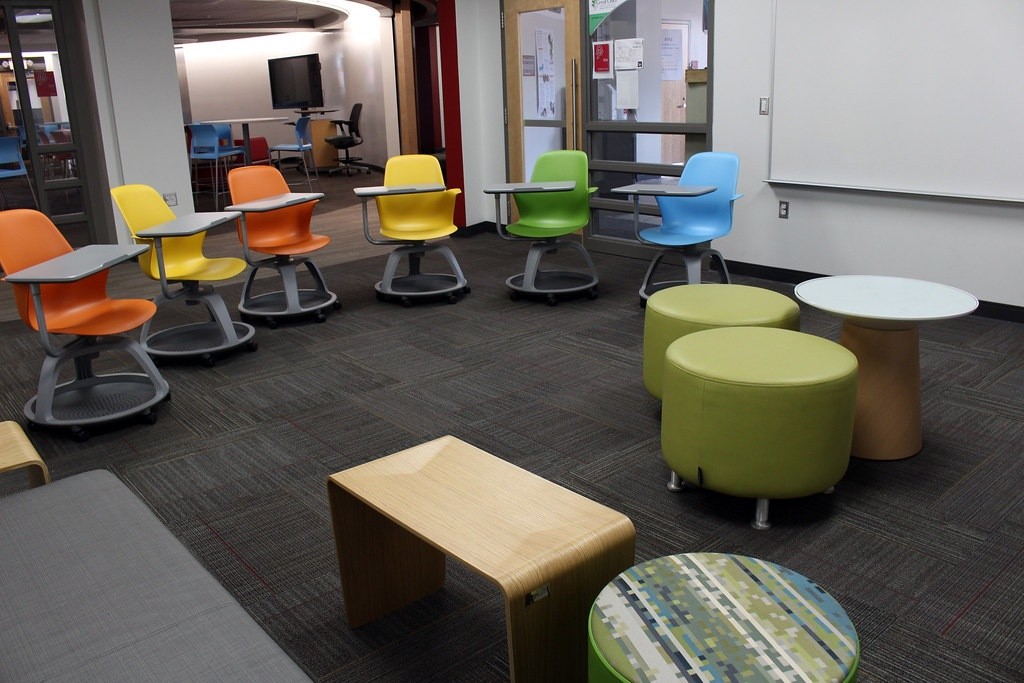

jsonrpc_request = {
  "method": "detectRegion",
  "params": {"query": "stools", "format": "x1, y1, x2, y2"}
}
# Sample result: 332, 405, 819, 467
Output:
326, 434, 637, 683
643, 283, 799, 399
587, 551, 860, 683
661, 326, 859, 530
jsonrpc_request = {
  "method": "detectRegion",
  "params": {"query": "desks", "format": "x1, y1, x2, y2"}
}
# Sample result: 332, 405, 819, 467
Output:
483, 180, 576, 241
199, 117, 288, 166
794, 275, 979, 460
224, 192, 325, 266
4, 244, 150, 357
611, 183, 717, 246
353, 184, 446, 246
136, 211, 243, 300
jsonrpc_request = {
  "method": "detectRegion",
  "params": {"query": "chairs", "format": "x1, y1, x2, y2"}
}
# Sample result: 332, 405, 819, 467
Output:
638, 151, 745, 306
110, 184, 259, 367
0, 207, 172, 444
506, 150, 603, 306
227, 165, 343, 329
324, 103, 372, 176
375, 154, 472, 309
0, 116, 318, 213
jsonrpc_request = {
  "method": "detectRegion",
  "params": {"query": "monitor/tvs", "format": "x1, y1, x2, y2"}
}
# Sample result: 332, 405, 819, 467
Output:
267, 54, 324, 118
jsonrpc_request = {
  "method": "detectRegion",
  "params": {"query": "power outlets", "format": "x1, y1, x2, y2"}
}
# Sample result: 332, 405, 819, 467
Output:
778, 200, 789, 219
